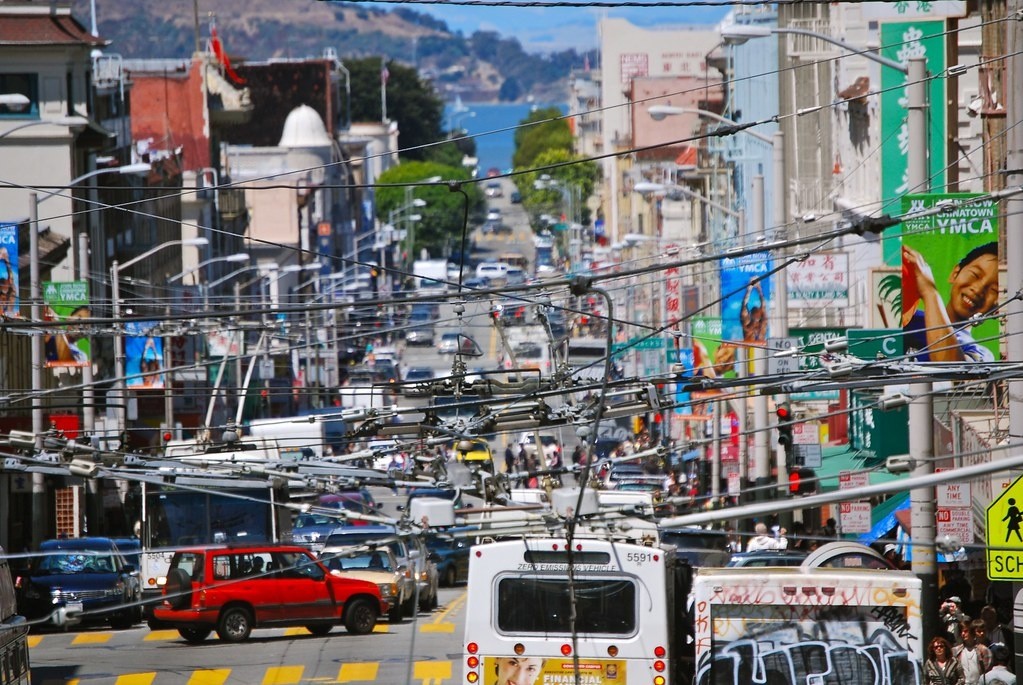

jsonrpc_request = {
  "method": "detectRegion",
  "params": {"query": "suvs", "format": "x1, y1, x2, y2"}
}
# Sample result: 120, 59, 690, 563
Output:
153, 545, 387, 646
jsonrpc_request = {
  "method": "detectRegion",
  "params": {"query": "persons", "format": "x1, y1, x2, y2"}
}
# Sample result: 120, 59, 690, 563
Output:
0, 247, 17, 313
140, 338, 161, 386
903, 241, 999, 362
741, 275, 767, 340
868, 542, 911, 570
250, 556, 265, 578
746, 518, 837, 564
46, 306, 90, 361
347, 331, 392, 380
924, 566, 1017, 685
428, 445, 449, 485
573, 419, 670, 485
503, 442, 567, 490
652, 471, 730, 530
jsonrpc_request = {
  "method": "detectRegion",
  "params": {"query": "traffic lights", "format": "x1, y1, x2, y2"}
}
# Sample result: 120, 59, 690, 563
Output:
787, 467, 814, 494
775, 402, 794, 448
162, 431, 173, 443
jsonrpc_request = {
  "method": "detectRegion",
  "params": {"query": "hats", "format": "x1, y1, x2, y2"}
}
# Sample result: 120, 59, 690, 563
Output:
945, 596, 961, 602
883, 544, 896, 556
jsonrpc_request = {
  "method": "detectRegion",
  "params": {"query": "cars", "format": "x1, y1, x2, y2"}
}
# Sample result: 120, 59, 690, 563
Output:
15, 535, 143, 626
240, 168, 818, 618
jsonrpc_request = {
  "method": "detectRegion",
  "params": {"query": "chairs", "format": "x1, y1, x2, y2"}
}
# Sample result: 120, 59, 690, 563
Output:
585, 596, 635, 635
266, 562, 274, 572
241, 562, 254, 576
498, 597, 550, 633
369, 560, 383, 569
328, 561, 342, 569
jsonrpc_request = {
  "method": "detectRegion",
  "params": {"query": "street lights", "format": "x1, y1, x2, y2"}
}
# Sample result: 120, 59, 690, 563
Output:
647, 106, 799, 501
201, 168, 445, 418
723, 25, 939, 658
108, 236, 212, 435
534, 182, 772, 490
162, 252, 251, 426
23, 160, 158, 559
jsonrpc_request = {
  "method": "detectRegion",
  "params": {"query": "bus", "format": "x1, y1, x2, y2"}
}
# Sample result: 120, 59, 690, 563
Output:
464, 482, 693, 685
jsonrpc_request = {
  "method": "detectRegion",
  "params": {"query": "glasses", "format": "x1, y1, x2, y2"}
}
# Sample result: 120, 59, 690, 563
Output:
934, 644, 944, 648
